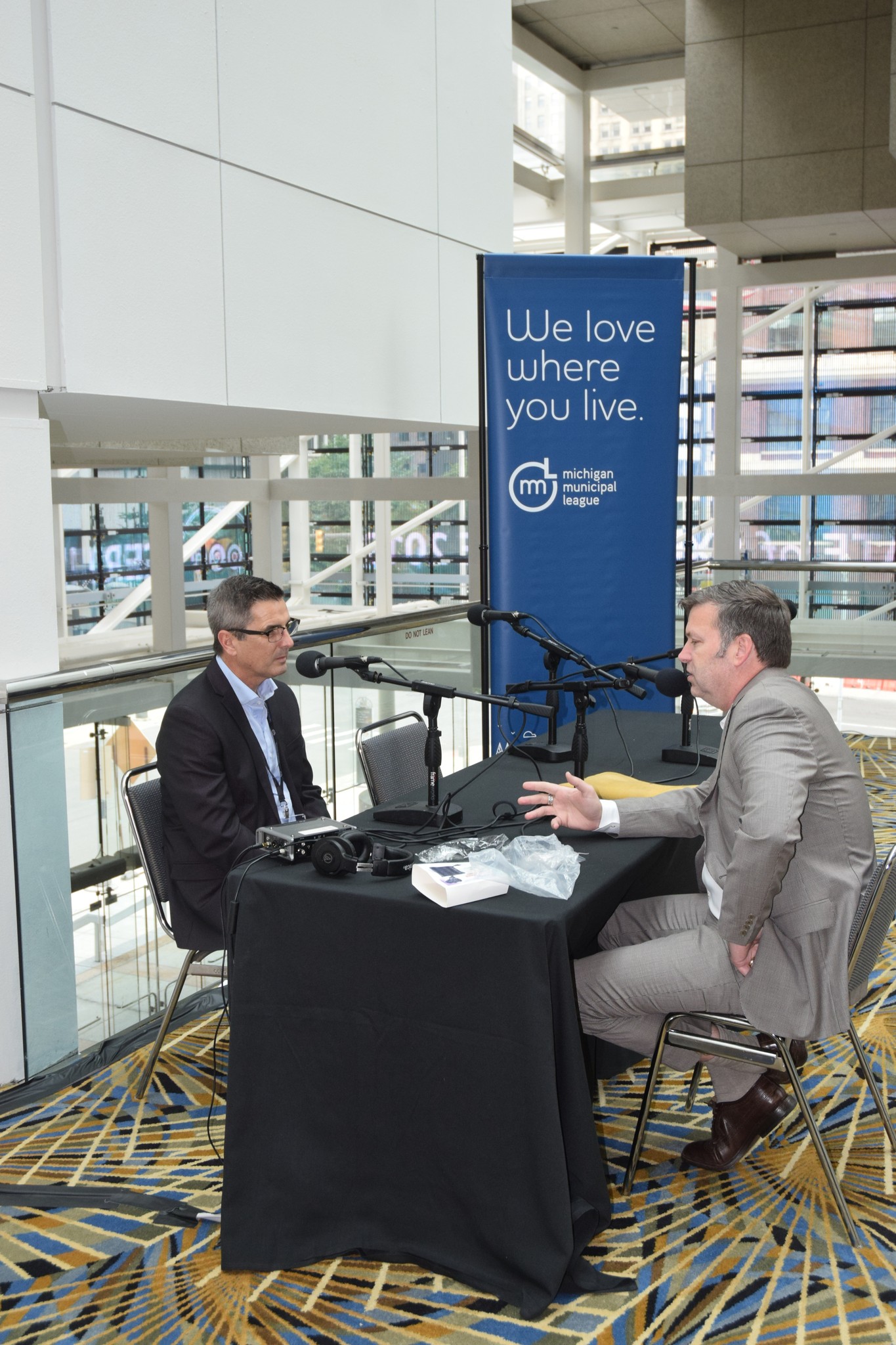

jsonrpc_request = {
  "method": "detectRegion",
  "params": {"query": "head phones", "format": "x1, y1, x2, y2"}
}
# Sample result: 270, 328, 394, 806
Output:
311, 830, 415, 877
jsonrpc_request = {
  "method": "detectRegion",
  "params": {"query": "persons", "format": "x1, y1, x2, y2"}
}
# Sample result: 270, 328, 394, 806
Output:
156, 576, 335, 949
515, 583, 878, 1172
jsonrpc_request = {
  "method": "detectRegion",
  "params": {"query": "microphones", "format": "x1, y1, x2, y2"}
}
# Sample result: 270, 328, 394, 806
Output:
295, 651, 381, 679
782, 598, 798, 620
617, 662, 688, 699
466, 603, 529, 626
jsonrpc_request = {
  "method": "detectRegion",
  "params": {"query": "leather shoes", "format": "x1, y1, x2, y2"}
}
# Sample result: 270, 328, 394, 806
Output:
681, 1075, 800, 1171
756, 1032, 807, 1085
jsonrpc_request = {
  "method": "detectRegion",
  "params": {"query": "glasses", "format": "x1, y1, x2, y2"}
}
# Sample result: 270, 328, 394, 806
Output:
227, 617, 302, 643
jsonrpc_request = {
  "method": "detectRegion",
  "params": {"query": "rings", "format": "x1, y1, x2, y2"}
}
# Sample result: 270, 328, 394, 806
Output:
548, 793, 553, 805
749, 959, 754, 966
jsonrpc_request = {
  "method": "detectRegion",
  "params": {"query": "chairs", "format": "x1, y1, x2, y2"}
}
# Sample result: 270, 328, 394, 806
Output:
121, 762, 227, 1099
355, 711, 443, 808
624, 844, 896, 1248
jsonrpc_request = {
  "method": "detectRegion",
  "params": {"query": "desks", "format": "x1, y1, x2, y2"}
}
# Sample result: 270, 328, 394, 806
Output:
221, 709, 726, 1321
